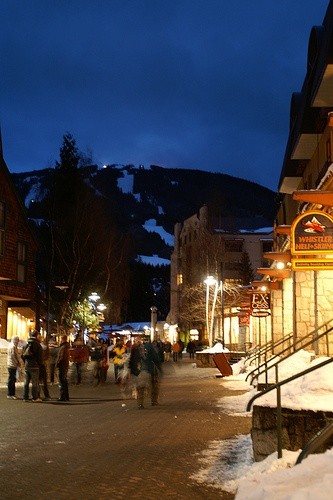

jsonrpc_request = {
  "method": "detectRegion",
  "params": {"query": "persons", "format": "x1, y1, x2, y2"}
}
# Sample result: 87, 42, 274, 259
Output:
172, 338, 196, 362
21, 330, 52, 402
6, 336, 22, 399
127, 339, 171, 408
56, 335, 69, 402
70, 337, 126, 387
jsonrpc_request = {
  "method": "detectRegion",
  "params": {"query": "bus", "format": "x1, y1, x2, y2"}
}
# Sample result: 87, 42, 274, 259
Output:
111, 330, 150, 354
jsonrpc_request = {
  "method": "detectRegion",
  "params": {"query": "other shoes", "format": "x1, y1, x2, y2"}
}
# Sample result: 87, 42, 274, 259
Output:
138, 406, 146, 410
24, 400, 30, 402
56, 397, 66, 402
7, 396, 18, 400
32, 397, 42, 402
152, 402, 158, 406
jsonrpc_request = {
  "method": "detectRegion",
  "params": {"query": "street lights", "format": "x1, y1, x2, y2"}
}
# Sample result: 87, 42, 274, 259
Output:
46, 280, 68, 357
203, 275, 225, 351
